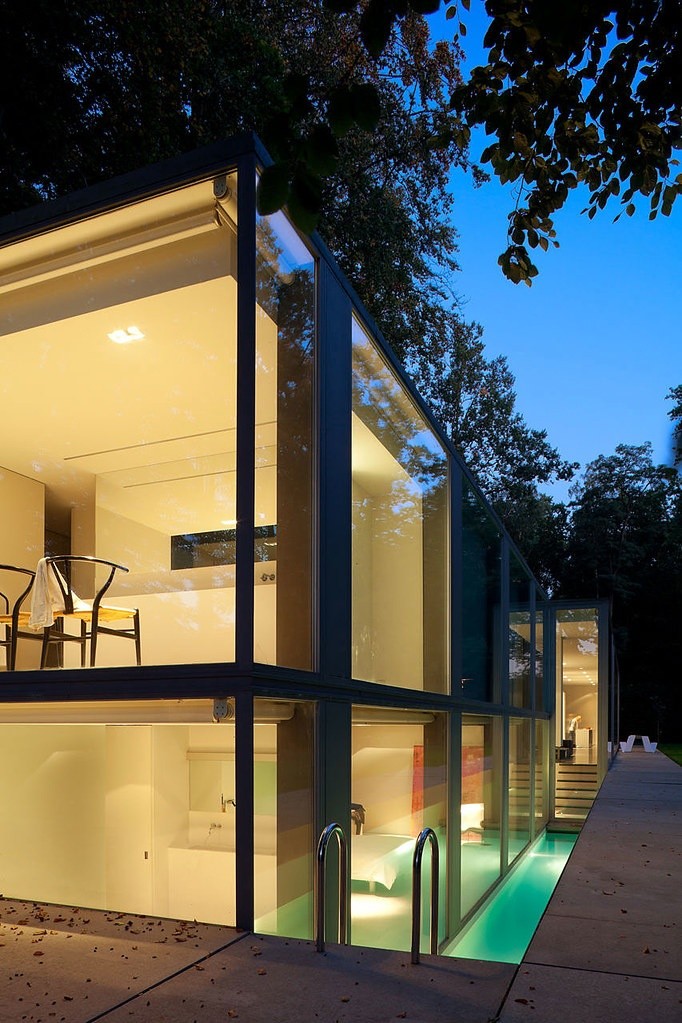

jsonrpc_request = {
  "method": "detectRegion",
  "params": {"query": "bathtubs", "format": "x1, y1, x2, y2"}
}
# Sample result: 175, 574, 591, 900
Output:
169, 843, 277, 926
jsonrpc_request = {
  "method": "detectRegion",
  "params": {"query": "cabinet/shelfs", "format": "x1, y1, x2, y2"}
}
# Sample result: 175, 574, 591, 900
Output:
575, 729, 593, 748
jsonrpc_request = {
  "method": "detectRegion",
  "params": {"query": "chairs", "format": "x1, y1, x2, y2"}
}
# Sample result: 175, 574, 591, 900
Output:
28, 555, 142, 669
0, 564, 66, 671
620, 735, 636, 752
641, 736, 656, 753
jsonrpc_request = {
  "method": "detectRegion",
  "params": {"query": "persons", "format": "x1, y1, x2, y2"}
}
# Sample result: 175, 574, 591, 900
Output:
566, 713, 581, 747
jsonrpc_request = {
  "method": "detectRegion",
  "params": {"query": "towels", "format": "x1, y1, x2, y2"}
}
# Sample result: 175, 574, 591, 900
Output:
28, 556, 92, 631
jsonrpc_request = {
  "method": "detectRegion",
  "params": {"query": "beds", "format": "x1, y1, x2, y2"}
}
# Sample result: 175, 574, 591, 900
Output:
351, 833, 417, 893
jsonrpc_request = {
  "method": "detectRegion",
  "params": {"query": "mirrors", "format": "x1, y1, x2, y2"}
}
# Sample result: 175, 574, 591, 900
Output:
186, 753, 276, 817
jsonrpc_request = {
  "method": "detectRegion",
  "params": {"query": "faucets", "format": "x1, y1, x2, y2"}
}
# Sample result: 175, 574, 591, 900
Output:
209, 823, 222, 835
